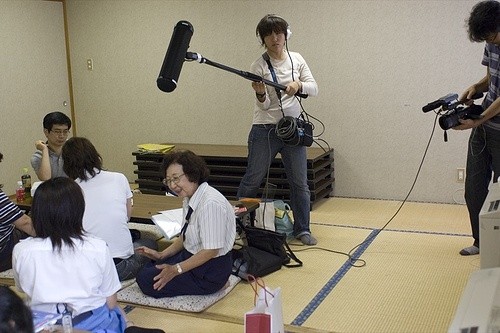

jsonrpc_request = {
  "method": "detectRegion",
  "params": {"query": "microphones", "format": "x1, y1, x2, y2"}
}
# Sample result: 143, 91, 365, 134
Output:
156, 20, 194, 93
421, 99, 447, 114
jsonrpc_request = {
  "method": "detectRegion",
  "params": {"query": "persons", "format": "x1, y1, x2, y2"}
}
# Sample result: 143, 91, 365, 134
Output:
11, 177, 127, 333
30, 111, 72, 181
234, 14, 320, 246
135, 149, 235, 298
0, 154, 36, 272
454, 0, 500, 255
61, 137, 157, 281
0, 286, 33, 333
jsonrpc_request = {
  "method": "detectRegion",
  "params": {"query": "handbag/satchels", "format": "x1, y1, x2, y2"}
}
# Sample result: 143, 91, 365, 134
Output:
230, 227, 303, 282
255, 201, 294, 243
243, 274, 285, 333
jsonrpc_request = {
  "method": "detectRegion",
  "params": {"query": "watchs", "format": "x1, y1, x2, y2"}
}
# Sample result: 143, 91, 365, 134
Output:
175, 262, 183, 274
296, 81, 300, 89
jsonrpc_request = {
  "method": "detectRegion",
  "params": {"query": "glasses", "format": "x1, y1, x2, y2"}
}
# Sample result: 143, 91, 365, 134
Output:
163, 174, 185, 185
486, 32, 498, 43
49, 130, 70, 136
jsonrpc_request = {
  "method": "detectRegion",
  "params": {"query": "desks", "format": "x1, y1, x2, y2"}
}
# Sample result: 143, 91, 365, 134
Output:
9, 184, 260, 228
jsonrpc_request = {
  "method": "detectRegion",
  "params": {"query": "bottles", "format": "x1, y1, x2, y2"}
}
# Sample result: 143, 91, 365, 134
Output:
16, 181, 24, 204
21, 168, 31, 199
